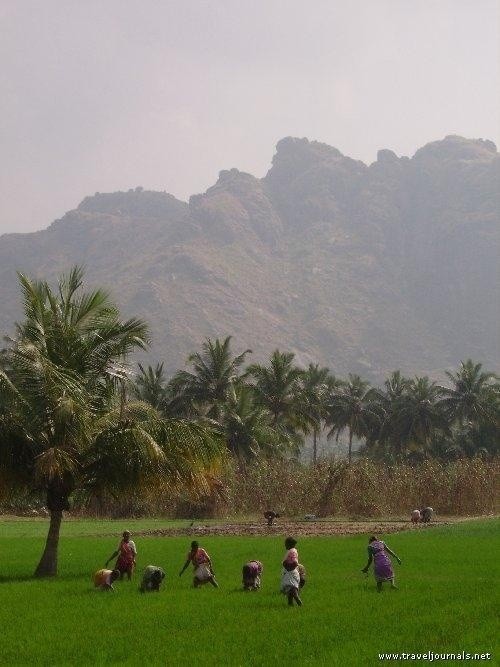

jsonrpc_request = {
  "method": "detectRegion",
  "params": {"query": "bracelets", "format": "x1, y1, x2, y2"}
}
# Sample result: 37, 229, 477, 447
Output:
397, 557, 400, 561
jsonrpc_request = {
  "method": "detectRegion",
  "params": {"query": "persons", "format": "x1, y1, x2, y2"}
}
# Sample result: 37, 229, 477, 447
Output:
140, 565, 165, 593
178, 539, 219, 586
411, 509, 420, 524
104, 529, 137, 580
280, 563, 307, 592
263, 509, 280, 525
360, 535, 401, 591
94, 568, 120, 590
420, 506, 433, 524
280, 536, 306, 608
243, 559, 263, 591
